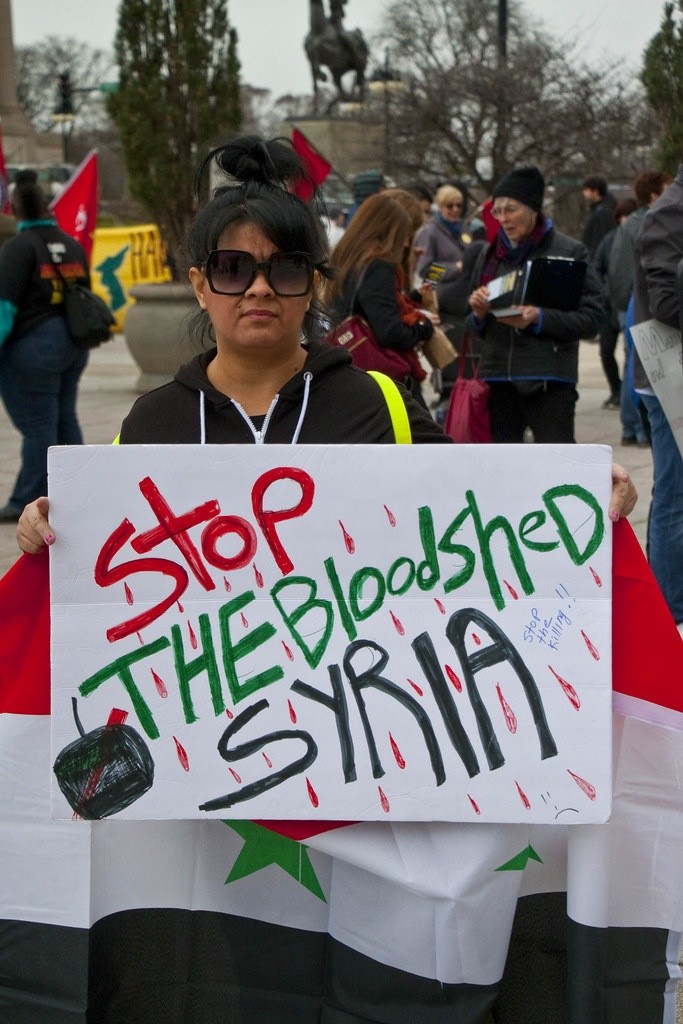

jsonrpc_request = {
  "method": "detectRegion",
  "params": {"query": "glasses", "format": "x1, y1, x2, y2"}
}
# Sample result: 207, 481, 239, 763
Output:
202, 248, 313, 297
490, 205, 529, 220
443, 203, 462, 209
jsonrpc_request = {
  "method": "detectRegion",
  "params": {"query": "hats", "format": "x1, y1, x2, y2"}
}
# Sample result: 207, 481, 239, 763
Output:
490, 164, 544, 213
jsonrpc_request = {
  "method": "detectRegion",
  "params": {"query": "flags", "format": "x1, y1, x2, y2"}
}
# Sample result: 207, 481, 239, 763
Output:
0, 512, 683, 1024
290, 130, 330, 202
48, 154, 98, 267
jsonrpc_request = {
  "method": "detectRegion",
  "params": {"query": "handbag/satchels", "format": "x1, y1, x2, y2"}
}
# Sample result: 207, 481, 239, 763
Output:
64, 284, 117, 351
322, 258, 427, 385
444, 330, 491, 443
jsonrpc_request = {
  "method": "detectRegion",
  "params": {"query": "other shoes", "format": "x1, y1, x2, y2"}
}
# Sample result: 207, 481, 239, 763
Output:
603, 394, 621, 409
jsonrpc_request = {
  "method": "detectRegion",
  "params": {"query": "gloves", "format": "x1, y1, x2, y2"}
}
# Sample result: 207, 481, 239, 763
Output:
408, 316, 435, 343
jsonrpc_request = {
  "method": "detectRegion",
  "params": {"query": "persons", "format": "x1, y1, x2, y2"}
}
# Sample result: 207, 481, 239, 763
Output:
627, 165, 683, 627
14, 138, 639, 555
308, 166, 676, 446
0, 168, 91, 523
